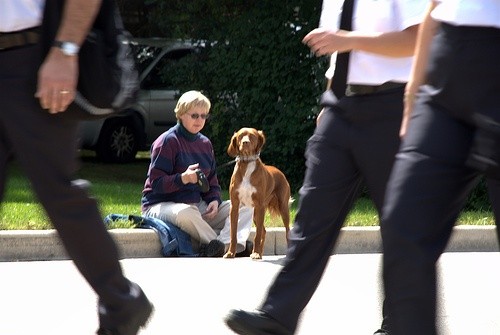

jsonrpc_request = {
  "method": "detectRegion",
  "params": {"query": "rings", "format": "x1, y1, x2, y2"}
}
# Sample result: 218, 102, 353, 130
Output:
61, 91, 69, 94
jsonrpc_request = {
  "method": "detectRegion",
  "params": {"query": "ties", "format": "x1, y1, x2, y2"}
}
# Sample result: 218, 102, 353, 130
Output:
331, 0, 354, 100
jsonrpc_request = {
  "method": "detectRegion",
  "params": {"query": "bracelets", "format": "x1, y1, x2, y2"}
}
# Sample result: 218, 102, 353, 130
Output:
404, 92, 415, 99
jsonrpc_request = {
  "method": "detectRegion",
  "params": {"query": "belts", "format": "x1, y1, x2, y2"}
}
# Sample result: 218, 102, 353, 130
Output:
0, 30, 39, 49
344, 82, 408, 97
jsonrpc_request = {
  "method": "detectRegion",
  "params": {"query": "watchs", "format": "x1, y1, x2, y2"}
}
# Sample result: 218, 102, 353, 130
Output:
52, 40, 79, 57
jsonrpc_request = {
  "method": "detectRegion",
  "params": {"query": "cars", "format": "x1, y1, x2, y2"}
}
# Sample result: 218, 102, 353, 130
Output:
75, 34, 239, 165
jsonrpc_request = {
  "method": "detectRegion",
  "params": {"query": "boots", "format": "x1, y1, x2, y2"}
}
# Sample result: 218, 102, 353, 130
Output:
35, 180, 153, 335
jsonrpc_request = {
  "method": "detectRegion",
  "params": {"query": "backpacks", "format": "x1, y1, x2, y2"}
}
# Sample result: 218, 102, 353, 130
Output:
42, 0, 137, 121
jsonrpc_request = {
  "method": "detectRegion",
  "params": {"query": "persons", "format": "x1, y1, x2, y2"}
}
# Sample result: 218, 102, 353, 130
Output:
140, 90, 254, 257
0, 0, 155, 335
227, 1, 438, 335
377, 0, 500, 335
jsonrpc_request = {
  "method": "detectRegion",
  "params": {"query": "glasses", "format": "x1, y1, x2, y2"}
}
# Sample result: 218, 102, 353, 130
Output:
186, 111, 208, 119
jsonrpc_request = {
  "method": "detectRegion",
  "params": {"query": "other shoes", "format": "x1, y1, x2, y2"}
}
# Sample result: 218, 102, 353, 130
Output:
199, 240, 224, 257
235, 240, 253, 257
222, 308, 296, 335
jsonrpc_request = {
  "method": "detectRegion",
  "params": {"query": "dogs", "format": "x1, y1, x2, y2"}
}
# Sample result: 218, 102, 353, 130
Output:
222, 127, 291, 259
